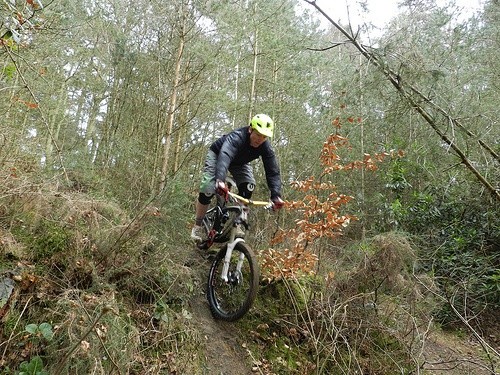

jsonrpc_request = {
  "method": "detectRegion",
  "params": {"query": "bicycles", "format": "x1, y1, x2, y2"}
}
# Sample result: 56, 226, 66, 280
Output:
196, 177, 276, 323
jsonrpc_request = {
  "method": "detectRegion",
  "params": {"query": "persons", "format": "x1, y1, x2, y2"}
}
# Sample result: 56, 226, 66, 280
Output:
191, 113, 287, 243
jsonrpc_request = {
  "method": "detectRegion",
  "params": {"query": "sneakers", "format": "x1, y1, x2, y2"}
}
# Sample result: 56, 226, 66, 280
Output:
234, 224, 246, 239
191, 223, 204, 243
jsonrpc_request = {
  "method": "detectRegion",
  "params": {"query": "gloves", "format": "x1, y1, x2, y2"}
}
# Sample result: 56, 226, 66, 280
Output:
271, 196, 286, 212
214, 179, 229, 203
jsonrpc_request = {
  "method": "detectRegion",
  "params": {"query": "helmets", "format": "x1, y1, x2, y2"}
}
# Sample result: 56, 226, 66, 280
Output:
250, 113, 275, 138
